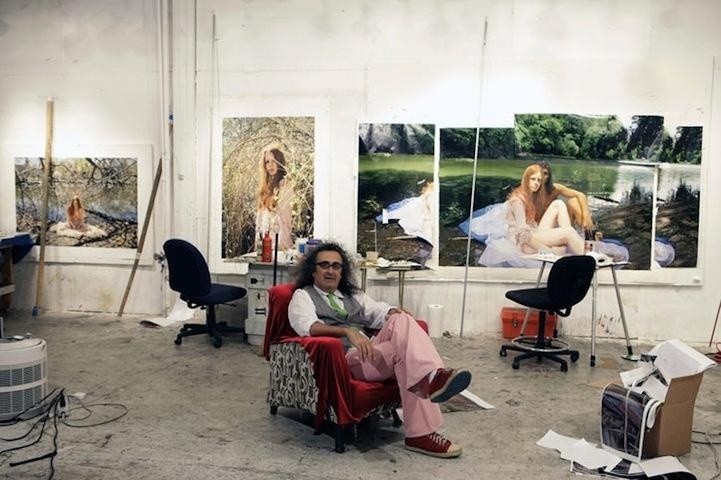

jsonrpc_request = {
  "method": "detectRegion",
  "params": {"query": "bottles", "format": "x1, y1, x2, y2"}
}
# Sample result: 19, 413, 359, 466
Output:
256, 231, 272, 262
295, 234, 323, 258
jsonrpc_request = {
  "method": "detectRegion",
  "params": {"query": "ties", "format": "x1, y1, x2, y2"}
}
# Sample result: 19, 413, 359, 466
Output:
326, 292, 348, 320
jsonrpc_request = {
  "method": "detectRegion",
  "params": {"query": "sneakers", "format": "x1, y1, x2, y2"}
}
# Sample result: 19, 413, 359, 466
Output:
404, 432, 464, 459
428, 367, 473, 403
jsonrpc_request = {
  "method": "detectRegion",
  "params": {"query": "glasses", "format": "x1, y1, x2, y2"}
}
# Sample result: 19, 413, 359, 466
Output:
316, 261, 343, 270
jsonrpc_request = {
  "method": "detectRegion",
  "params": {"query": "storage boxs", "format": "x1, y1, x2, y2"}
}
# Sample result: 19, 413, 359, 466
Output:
500, 306, 556, 340
608, 371, 703, 459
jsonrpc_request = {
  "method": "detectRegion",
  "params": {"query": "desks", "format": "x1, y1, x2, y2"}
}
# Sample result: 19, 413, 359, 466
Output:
356, 261, 412, 312
0, 230, 34, 313
518, 254, 633, 369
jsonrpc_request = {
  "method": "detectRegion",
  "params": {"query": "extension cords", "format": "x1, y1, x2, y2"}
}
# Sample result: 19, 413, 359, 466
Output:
56, 396, 69, 418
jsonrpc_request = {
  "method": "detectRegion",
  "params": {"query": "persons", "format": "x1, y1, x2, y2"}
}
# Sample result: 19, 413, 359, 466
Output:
286, 242, 472, 458
252, 147, 294, 249
538, 161, 595, 235
66, 195, 89, 232
507, 166, 589, 257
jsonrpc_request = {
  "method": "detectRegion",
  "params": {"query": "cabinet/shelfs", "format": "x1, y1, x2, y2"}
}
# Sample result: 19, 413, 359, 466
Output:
244, 259, 350, 345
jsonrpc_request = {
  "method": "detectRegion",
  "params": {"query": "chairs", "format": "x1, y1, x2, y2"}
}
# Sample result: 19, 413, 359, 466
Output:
266, 281, 428, 454
499, 255, 596, 373
163, 238, 247, 348
